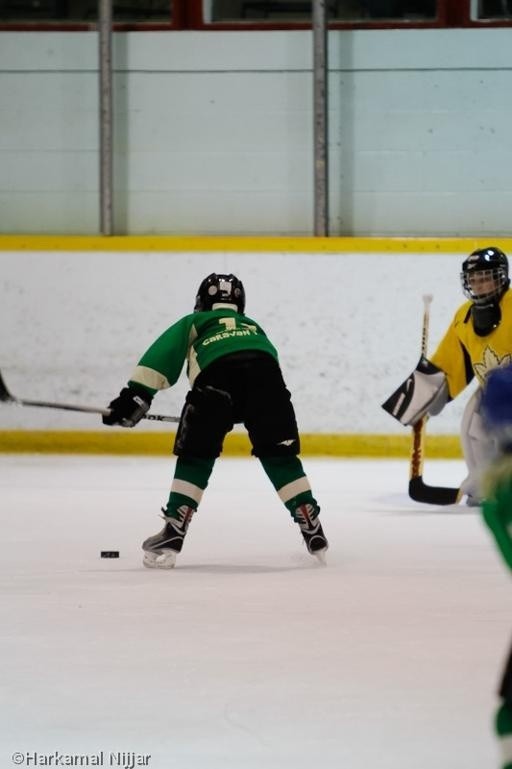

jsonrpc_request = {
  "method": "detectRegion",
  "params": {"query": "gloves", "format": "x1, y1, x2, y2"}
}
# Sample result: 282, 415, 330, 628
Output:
102, 388, 152, 425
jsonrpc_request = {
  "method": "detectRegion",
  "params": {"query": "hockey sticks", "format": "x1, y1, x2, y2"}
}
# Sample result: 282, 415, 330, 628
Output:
408, 291, 463, 504
0, 372, 181, 423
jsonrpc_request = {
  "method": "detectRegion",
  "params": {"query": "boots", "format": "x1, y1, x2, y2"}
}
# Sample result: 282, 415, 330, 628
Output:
143, 501, 195, 569
294, 498, 327, 561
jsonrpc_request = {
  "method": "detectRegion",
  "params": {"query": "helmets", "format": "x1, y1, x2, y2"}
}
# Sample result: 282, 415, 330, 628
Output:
193, 270, 246, 315
459, 245, 511, 310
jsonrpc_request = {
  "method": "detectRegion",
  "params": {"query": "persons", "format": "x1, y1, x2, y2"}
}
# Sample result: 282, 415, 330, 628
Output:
381, 248, 511, 508
102, 273, 329, 568
481, 366, 511, 769
